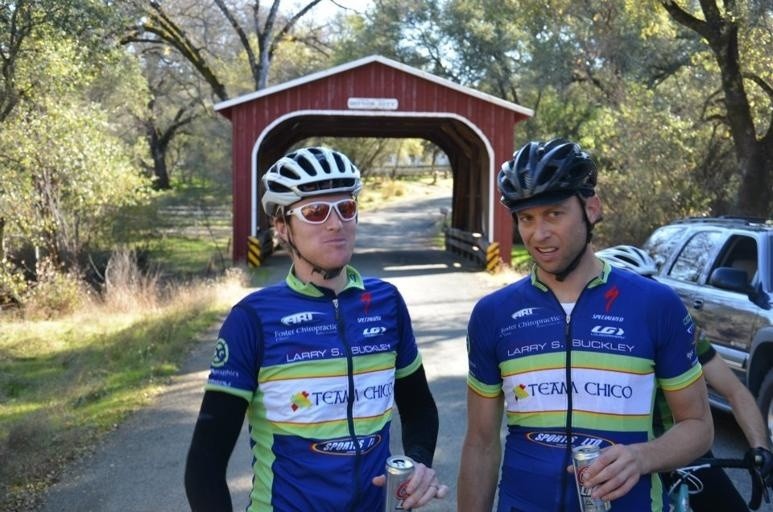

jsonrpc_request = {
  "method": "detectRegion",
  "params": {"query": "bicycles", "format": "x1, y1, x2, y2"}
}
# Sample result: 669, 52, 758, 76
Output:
670, 447, 771, 512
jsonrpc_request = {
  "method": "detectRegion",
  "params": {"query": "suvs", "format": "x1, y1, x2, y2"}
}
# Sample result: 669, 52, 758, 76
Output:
642, 213, 773, 445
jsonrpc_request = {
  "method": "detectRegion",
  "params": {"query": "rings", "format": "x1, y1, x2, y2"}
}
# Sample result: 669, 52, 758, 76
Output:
427, 484, 440, 493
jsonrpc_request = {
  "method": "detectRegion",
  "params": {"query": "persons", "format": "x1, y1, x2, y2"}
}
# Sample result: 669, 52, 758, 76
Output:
595, 245, 773, 512
458, 138, 715, 512
185, 147, 450, 512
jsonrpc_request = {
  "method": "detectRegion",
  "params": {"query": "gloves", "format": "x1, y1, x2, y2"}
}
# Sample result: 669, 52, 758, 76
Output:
743, 445, 772, 506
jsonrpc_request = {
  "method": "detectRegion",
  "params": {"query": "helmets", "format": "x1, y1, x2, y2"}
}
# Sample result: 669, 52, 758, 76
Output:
258, 146, 362, 215
495, 140, 598, 209
591, 241, 660, 277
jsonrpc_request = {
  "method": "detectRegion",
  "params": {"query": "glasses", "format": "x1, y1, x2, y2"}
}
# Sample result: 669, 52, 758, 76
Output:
285, 203, 359, 224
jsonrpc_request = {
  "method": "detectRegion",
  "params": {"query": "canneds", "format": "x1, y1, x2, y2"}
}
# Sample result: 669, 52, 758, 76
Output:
571, 445, 611, 512
385, 455, 415, 512
